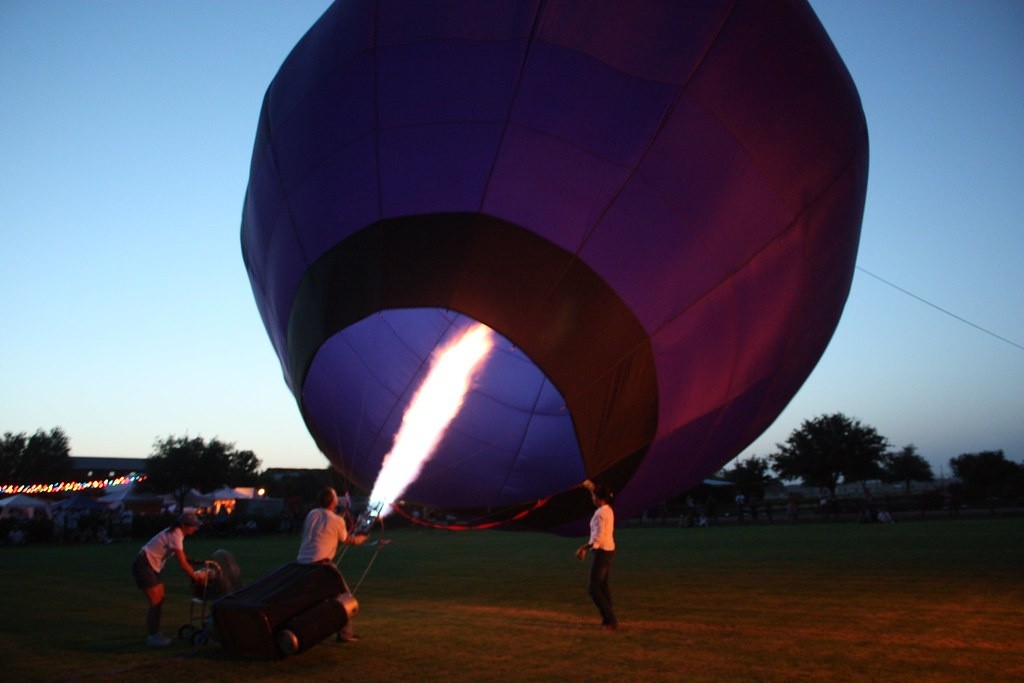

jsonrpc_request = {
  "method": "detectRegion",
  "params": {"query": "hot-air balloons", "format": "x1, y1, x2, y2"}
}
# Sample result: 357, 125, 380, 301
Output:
199, 0, 876, 661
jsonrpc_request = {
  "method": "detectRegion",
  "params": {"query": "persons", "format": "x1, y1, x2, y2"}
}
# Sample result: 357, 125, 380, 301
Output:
920, 494, 928, 516
135, 513, 213, 646
576, 479, 618, 628
8, 501, 311, 546
784, 491, 799, 522
296, 488, 369, 642
734, 489, 773, 522
943, 487, 953, 517
679, 508, 709, 528
822, 493, 896, 525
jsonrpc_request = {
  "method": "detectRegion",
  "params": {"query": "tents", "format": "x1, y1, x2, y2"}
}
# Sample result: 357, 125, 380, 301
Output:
0, 487, 286, 516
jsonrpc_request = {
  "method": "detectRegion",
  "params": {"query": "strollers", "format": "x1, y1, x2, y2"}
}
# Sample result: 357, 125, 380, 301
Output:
176, 550, 249, 646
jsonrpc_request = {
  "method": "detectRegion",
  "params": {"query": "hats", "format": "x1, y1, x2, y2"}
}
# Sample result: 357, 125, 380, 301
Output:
179, 513, 203, 526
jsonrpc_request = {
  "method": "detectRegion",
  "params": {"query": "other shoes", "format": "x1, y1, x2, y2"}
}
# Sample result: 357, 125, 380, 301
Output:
147, 632, 172, 648
336, 635, 358, 643
599, 622, 619, 630
